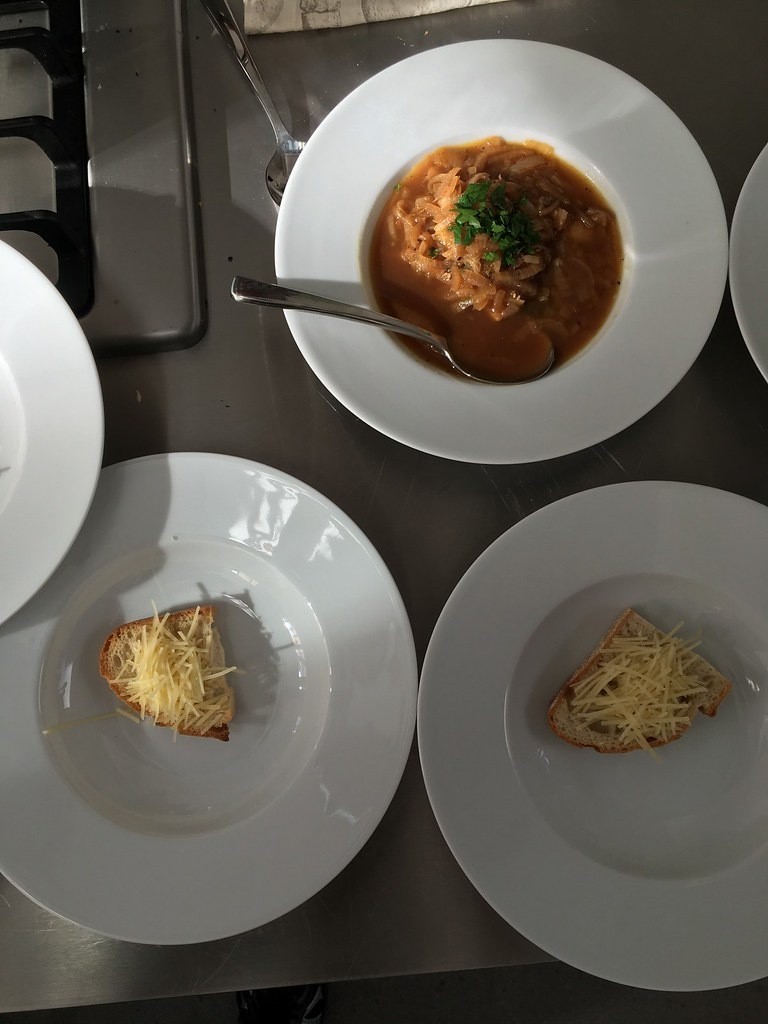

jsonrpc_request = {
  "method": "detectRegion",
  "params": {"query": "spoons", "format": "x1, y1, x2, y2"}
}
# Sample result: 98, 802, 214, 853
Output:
204, 0, 308, 210
230, 276, 555, 386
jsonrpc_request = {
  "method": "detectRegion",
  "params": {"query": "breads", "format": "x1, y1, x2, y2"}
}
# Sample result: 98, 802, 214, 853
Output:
546, 607, 732, 752
98, 605, 234, 740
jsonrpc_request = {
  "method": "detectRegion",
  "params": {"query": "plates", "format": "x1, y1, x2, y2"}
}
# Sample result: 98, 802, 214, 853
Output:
273, 41, 728, 464
1, 451, 418, 947
727, 142, 768, 386
0, 240, 107, 625
416, 482, 767, 993
